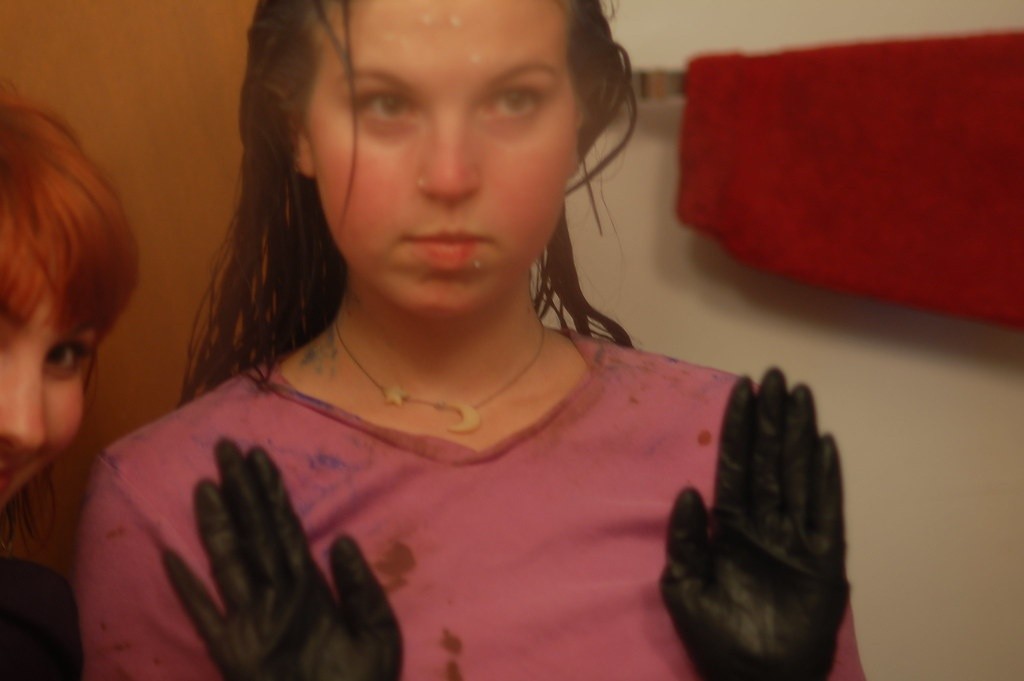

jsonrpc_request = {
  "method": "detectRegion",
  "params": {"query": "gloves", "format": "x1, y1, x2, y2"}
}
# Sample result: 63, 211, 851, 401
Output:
160, 438, 402, 681
660, 366, 850, 681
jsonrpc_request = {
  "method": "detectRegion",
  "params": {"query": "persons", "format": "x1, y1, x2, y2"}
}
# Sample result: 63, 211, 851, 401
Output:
75, 0, 868, 681
0, 79, 140, 681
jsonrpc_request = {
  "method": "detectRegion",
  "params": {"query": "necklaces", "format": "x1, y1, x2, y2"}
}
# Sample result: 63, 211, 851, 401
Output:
333, 316, 546, 432
